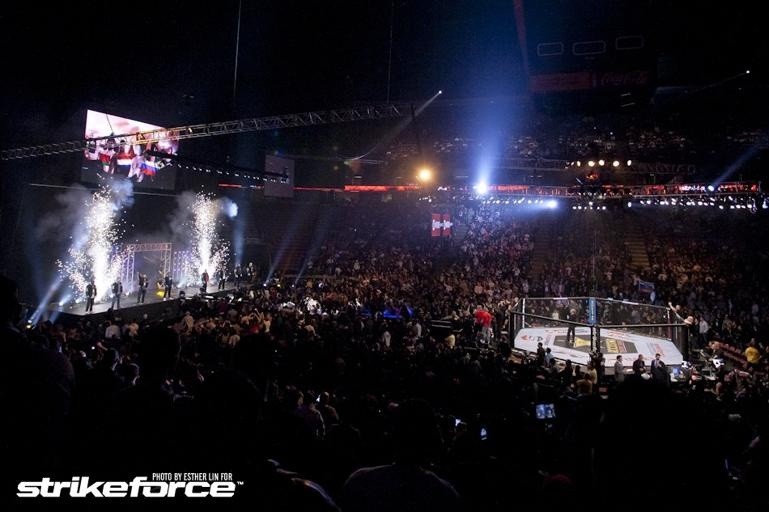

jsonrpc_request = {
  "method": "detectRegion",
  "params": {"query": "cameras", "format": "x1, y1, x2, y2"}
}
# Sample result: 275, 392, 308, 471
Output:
536, 403, 557, 419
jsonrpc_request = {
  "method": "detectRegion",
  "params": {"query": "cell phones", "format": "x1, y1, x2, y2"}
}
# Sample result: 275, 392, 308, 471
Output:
455, 419, 461, 427
480, 428, 487, 440
316, 394, 321, 402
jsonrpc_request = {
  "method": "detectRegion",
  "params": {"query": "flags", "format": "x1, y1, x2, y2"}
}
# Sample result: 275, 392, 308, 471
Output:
96, 151, 157, 180
